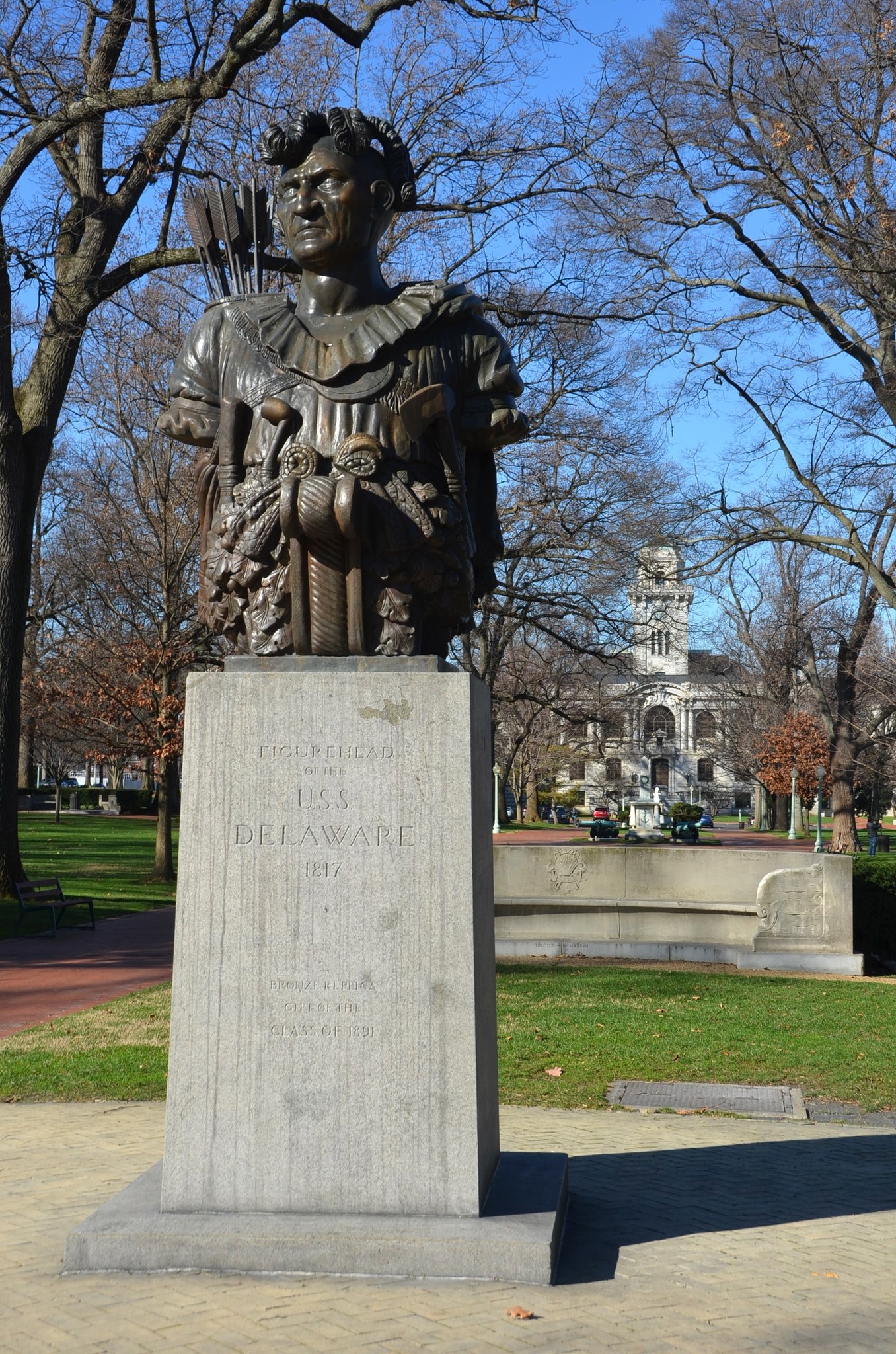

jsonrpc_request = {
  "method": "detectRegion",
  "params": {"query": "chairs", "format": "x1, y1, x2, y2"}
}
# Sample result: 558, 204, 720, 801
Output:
817, 841, 834, 853
832, 844, 848, 855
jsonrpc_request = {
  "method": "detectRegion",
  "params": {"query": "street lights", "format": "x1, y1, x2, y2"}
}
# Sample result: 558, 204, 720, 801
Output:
813, 763, 827, 853
787, 766, 799, 840
492, 762, 502, 834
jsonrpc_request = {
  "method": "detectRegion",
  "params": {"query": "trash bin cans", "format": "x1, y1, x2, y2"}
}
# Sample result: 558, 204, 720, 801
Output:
70, 794, 80, 810
739, 822, 745, 829
573, 819, 579, 825
877, 835, 891, 852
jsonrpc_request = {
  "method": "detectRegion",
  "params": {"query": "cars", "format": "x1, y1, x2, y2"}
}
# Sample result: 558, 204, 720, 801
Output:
747, 813, 755, 827
699, 815, 715, 828
506, 806, 515, 819
538, 806, 552, 821
522, 805, 527, 818
36, 777, 104, 789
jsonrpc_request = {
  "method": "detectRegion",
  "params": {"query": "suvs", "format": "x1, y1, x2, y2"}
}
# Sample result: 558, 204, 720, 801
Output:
592, 806, 611, 821
548, 805, 571, 824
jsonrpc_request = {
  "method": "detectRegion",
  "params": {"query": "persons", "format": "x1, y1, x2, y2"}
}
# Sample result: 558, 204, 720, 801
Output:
156, 109, 530, 654
571, 807, 577, 822
867, 815, 882, 856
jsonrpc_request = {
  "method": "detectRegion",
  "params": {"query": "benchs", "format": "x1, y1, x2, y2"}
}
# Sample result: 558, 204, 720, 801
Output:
14, 875, 96, 938
